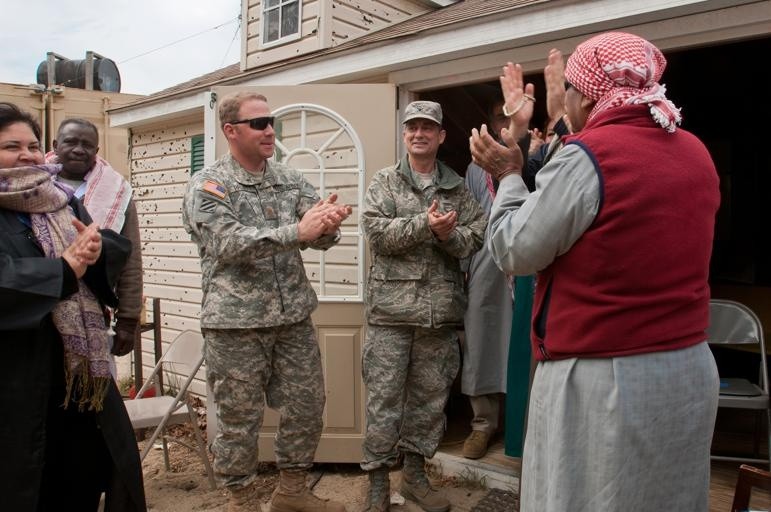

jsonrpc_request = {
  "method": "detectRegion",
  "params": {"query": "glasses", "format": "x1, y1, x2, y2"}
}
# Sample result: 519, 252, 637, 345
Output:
234, 116, 277, 129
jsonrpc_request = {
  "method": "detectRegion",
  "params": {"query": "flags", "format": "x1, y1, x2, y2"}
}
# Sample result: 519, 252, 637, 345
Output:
203, 180, 227, 198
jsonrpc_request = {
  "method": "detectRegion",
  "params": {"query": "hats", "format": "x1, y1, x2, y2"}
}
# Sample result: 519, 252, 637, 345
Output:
401, 101, 442, 124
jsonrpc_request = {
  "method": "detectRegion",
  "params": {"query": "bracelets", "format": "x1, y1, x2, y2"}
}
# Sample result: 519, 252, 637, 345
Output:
502, 94, 536, 117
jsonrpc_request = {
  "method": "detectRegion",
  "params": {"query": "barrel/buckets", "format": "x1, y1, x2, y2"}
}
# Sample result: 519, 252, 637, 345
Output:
36, 57, 121, 93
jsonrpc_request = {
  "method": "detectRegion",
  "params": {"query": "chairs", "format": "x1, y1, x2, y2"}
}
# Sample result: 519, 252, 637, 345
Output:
121, 329, 218, 489
704, 299, 771, 466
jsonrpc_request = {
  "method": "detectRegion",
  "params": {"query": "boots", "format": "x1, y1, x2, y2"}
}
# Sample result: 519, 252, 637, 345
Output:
363, 469, 391, 512
267, 470, 346, 512
399, 453, 450, 512
230, 484, 261, 512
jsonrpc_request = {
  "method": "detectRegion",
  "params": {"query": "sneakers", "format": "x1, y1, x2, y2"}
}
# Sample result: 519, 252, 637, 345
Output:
463, 432, 490, 459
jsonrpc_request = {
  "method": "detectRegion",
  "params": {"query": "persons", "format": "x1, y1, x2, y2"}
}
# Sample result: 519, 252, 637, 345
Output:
459, 95, 513, 459
359, 101, 488, 512
470, 30, 721, 512
43, 116, 143, 357
499, 48, 578, 458
528, 120, 556, 160
181, 92, 352, 512
0, 102, 133, 512
